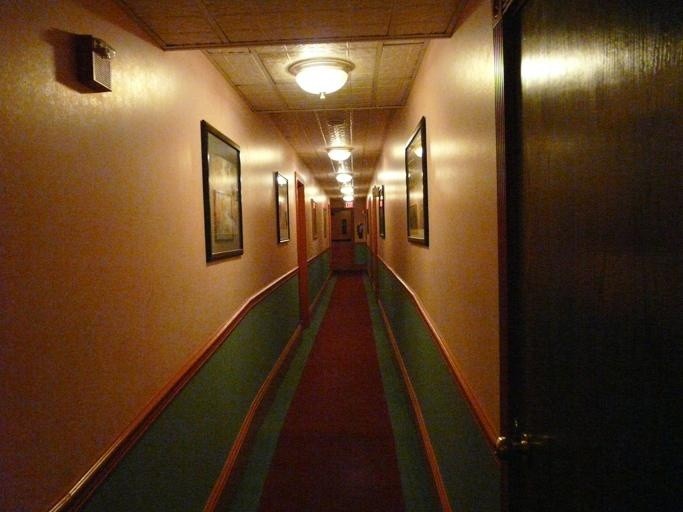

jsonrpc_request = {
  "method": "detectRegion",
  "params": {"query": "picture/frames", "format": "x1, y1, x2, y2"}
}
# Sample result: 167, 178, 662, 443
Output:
310, 198, 327, 240
405, 116, 429, 247
275, 171, 291, 243
199, 119, 244, 263
378, 185, 385, 239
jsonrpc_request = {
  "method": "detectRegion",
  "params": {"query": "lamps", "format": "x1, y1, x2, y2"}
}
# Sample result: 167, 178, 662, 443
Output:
322, 117, 360, 203
287, 57, 356, 100
78, 33, 116, 94
